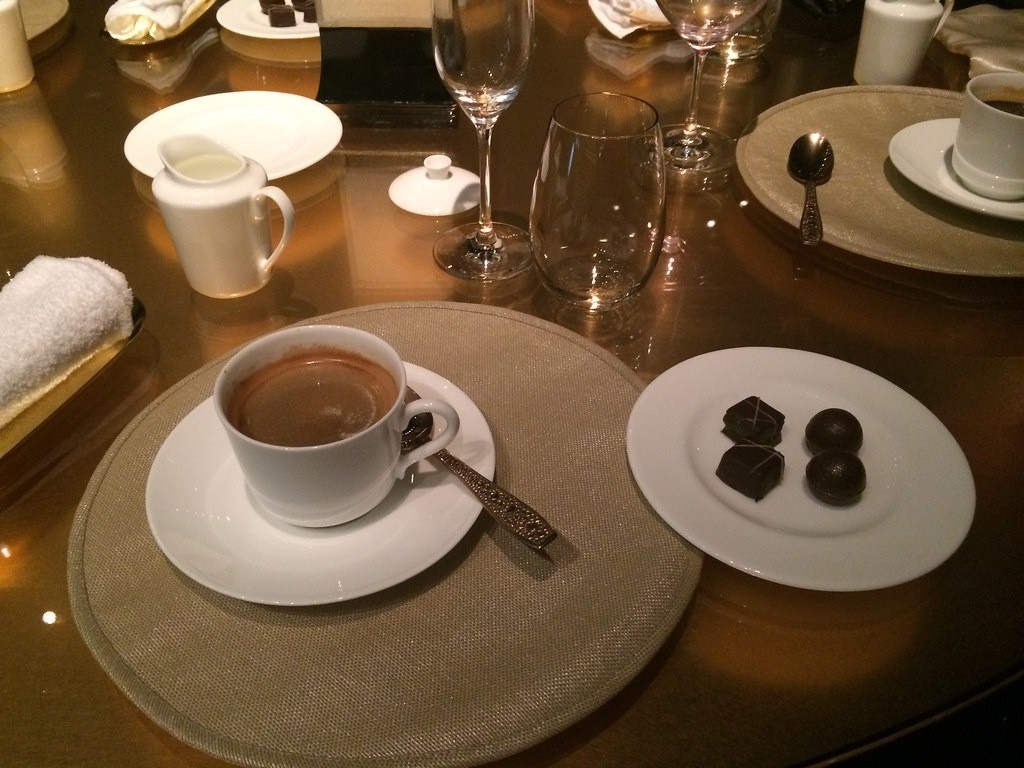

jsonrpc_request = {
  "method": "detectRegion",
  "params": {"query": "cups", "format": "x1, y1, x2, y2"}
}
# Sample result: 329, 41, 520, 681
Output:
529, 92, 665, 311
706, 0, 782, 65
952, 73, 1024, 201
214, 325, 459, 527
151, 134, 295, 299
0, 0, 34, 94
854, 0, 955, 85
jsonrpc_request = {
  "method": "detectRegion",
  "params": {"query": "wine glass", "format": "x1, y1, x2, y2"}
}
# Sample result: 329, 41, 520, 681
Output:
655, 0, 768, 173
433, 0, 535, 283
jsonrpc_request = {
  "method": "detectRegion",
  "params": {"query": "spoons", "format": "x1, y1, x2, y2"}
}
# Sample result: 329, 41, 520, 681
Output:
401, 385, 558, 550
787, 133, 835, 246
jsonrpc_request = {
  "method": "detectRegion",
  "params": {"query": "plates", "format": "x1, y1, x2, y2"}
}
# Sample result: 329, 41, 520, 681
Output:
124, 91, 343, 181
0, 295, 147, 460
20, 0, 69, 40
216, 0, 321, 39
626, 347, 975, 592
889, 118, 1024, 220
145, 361, 495, 605
117, 0, 217, 45
